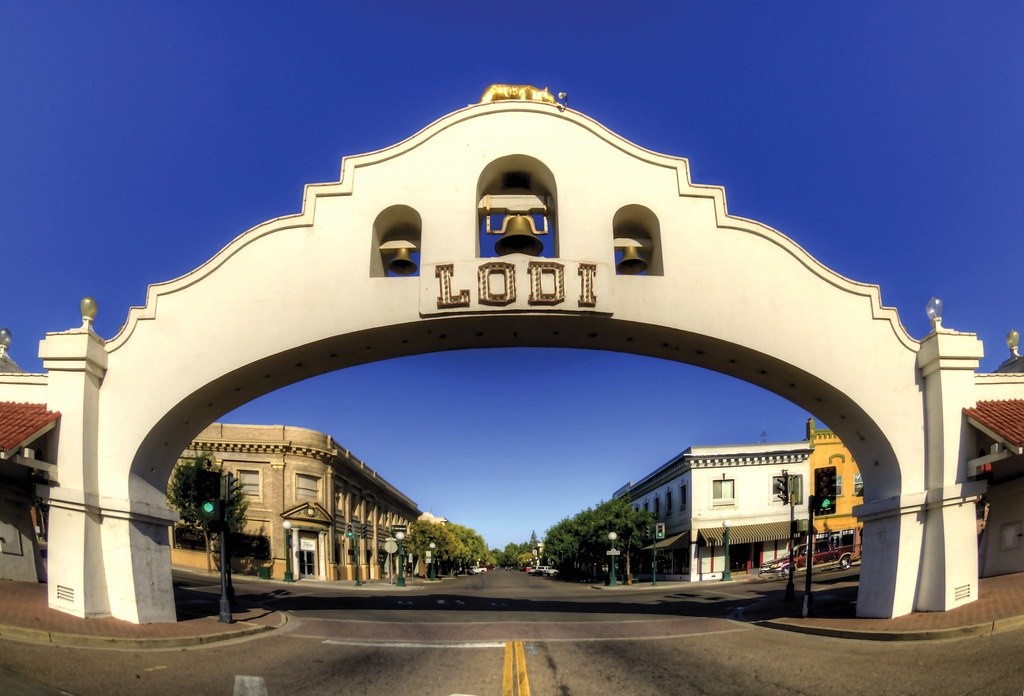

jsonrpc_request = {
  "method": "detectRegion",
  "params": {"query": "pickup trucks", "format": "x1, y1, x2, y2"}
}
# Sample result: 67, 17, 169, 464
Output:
528, 566, 559, 576
468, 566, 487, 574
759, 540, 856, 579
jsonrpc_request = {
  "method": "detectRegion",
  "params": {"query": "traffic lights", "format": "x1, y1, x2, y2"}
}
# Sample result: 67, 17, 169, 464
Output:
656, 523, 665, 539
346, 523, 353, 537
777, 476, 789, 506
645, 526, 652, 540
347, 549, 357, 556
196, 469, 220, 520
361, 524, 367, 538
814, 466, 837, 516
656, 550, 663, 556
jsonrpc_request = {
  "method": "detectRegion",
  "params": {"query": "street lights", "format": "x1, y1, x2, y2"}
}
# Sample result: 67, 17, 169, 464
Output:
430, 542, 436, 580
605, 532, 617, 586
396, 531, 406, 586
283, 520, 295, 582
721, 520, 733, 581
